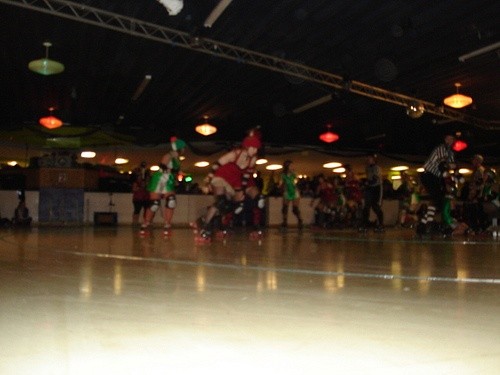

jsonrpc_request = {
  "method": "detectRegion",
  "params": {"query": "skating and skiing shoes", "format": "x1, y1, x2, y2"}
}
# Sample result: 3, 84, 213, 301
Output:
164, 224, 169, 236
358, 227, 369, 233
141, 225, 146, 234
195, 228, 212, 242
220, 225, 233, 238
374, 226, 385, 234
254, 225, 264, 239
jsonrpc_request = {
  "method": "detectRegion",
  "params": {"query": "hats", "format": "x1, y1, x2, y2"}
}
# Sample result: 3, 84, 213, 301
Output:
244, 138, 260, 148
171, 137, 186, 151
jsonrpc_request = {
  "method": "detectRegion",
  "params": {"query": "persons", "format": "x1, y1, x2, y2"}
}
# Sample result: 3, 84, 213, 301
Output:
275, 161, 304, 235
190, 135, 261, 243
358, 151, 386, 235
467, 153, 485, 204
415, 134, 457, 242
309, 175, 364, 227
133, 160, 154, 229
439, 196, 500, 241
395, 170, 416, 231
215, 164, 263, 239
140, 138, 187, 238
